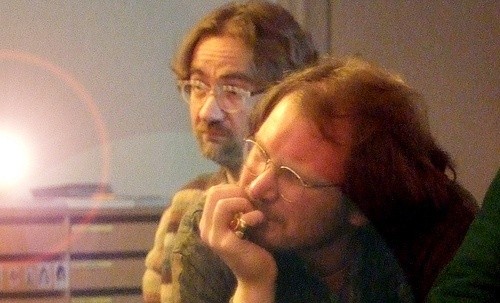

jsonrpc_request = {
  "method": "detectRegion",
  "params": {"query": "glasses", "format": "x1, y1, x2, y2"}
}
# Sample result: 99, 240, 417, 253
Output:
242, 133, 340, 204
181, 80, 265, 114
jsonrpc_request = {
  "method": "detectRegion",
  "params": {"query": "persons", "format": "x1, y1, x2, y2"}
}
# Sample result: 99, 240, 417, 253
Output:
141, 0, 319, 303
424, 167, 500, 302
171, 62, 471, 303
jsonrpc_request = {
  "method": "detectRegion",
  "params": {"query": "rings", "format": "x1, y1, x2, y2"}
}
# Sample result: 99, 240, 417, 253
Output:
229, 217, 251, 240
